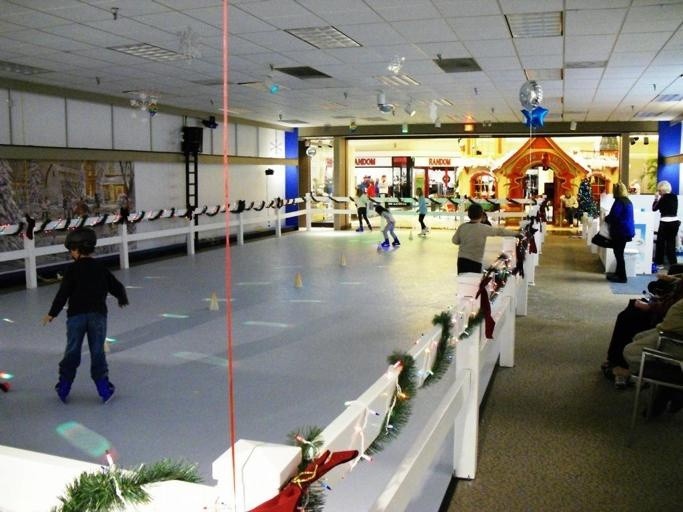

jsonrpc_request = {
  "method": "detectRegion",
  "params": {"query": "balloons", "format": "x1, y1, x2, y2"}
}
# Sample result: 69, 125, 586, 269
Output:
518, 80, 544, 112
519, 105, 549, 129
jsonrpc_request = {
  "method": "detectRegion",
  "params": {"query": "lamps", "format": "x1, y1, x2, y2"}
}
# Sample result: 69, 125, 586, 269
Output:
346, 88, 578, 136
263, 75, 279, 95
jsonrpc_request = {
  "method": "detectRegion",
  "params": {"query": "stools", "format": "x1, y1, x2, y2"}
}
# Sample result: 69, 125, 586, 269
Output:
628, 327, 682, 431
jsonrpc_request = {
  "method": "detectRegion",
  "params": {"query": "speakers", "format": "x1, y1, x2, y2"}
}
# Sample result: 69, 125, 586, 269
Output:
183, 127, 203, 153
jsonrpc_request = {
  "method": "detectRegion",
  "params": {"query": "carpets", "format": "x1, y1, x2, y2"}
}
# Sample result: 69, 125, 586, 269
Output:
608, 274, 662, 294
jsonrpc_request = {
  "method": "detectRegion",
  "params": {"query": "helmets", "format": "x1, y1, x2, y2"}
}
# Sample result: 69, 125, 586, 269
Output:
65, 226, 96, 249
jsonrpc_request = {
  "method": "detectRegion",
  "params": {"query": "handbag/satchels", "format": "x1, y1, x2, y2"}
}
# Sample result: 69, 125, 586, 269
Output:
591, 220, 611, 248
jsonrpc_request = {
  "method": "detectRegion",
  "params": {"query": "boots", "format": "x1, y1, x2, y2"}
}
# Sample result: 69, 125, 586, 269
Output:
392, 238, 398, 244
356, 226, 362, 231
381, 239, 389, 245
94, 378, 114, 399
55, 379, 73, 400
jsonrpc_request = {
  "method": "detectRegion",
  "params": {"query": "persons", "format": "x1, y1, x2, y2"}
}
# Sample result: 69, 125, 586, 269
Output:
40, 226, 129, 403
374, 206, 400, 247
39, 192, 131, 220
354, 188, 372, 232
599, 264, 682, 417
559, 190, 578, 228
415, 188, 430, 235
310, 173, 406, 198
452, 203, 524, 278
603, 182, 635, 284
651, 180, 680, 266
479, 211, 491, 226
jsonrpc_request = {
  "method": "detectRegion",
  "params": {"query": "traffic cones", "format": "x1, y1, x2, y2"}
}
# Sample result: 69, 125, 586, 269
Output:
339, 252, 347, 266
207, 291, 220, 312
103, 340, 110, 353
406, 229, 414, 240
292, 271, 304, 289
